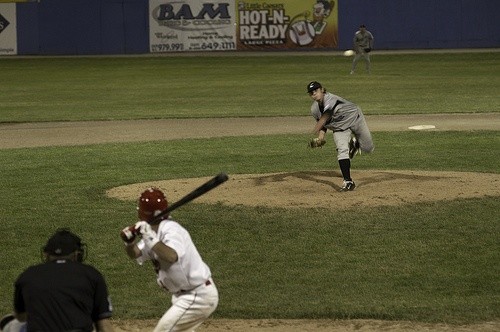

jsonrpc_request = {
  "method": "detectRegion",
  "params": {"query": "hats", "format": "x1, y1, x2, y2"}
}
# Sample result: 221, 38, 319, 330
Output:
307, 81, 320, 93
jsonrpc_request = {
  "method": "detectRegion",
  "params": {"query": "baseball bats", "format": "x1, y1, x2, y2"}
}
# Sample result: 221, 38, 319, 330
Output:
121, 172, 229, 244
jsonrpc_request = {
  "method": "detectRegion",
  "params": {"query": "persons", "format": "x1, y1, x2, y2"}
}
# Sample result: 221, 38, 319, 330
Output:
121, 188, 218, 332
306, 81, 375, 193
350, 25, 374, 75
13, 228, 114, 332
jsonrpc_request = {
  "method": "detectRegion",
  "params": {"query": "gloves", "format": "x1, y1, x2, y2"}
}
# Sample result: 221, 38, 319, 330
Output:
135, 222, 160, 251
121, 226, 136, 249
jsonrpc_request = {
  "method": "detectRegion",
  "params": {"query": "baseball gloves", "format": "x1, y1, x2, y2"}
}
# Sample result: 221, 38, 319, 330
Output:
364, 48, 372, 53
308, 137, 325, 148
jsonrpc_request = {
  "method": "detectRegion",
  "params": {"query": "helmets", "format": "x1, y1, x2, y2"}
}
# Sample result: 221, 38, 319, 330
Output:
44, 230, 83, 262
138, 188, 168, 224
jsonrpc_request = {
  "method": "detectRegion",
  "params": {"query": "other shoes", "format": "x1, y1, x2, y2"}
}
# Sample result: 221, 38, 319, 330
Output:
340, 179, 356, 192
348, 136, 361, 159
